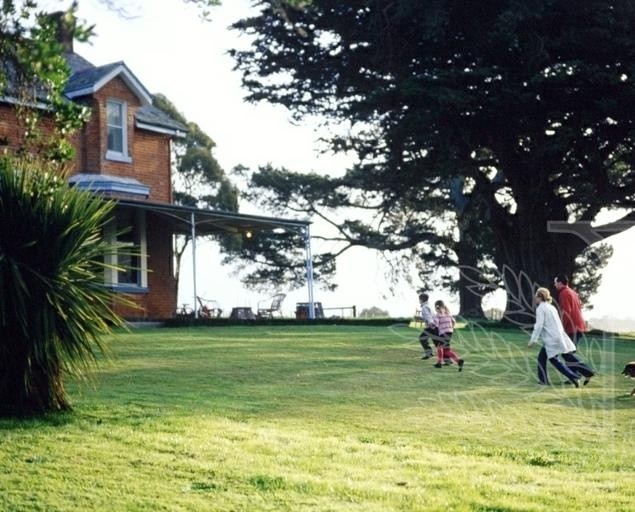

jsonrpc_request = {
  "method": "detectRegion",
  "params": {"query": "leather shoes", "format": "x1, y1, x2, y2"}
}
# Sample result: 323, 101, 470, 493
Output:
565, 375, 590, 388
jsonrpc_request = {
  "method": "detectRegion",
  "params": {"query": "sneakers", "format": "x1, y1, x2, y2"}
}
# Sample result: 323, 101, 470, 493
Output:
422, 354, 464, 372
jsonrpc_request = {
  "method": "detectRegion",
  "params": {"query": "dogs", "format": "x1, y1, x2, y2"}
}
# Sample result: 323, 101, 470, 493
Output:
621, 362, 635, 395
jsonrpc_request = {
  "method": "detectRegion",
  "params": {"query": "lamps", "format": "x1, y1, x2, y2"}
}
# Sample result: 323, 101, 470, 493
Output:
246, 232, 252, 238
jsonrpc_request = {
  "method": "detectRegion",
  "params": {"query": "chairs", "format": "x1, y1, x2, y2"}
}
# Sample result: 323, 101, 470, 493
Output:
257, 294, 286, 319
197, 296, 220, 318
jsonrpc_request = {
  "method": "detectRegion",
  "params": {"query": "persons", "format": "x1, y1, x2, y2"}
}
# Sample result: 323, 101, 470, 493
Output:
553, 273, 593, 386
528, 287, 580, 389
429, 300, 464, 372
419, 294, 453, 365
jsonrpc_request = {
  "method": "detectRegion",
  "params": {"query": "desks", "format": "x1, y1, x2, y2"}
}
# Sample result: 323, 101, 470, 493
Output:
230, 307, 257, 320
